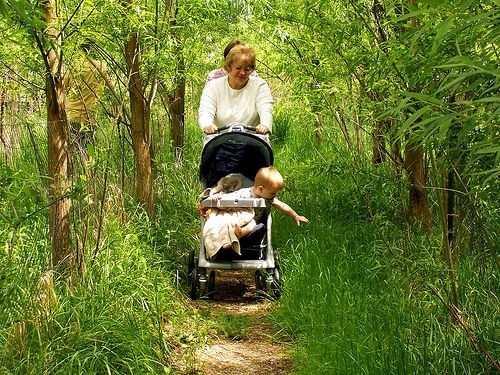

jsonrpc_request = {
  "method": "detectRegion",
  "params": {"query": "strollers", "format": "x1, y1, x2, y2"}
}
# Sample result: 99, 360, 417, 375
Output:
188, 122, 280, 300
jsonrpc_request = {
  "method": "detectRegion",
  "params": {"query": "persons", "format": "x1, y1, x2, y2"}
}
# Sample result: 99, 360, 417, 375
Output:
212, 165, 310, 249
205, 40, 259, 85
197, 44, 274, 154
